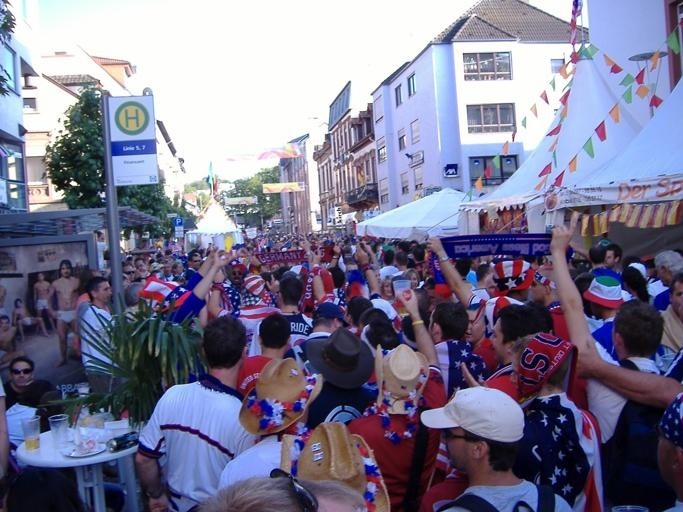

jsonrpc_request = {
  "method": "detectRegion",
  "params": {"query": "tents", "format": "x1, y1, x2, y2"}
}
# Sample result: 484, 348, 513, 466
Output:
548, 76, 682, 215
461, 41, 651, 214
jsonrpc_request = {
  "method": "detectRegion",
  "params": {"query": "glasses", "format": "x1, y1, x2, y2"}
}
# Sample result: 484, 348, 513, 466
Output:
269, 467, 321, 508
136, 263, 146, 269
123, 271, 137, 275
10, 367, 35, 376
193, 259, 203, 263
232, 269, 244, 276
444, 428, 467, 444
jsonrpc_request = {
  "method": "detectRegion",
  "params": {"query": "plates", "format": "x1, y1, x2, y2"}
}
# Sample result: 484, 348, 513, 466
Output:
61, 442, 106, 457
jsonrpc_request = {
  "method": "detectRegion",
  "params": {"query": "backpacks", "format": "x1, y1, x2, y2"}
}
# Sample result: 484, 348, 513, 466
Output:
449, 484, 555, 512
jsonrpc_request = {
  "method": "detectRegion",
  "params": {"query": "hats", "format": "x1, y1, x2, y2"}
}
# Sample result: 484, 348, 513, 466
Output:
583, 275, 627, 311
376, 342, 431, 416
304, 328, 376, 389
628, 261, 648, 281
493, 260, 535, 298
280, 420, 391, 511
316, 300, 350, 327
149, 262, 165, 273
238, 357, 323, 436
372, 296, 400, 320
420, 384, 526, 444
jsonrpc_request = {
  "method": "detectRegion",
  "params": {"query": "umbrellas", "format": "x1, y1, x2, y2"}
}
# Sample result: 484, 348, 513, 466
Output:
355, 187, 480, 242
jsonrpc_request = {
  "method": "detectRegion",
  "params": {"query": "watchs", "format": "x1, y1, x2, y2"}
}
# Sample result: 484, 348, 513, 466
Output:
438, 256, 450, 262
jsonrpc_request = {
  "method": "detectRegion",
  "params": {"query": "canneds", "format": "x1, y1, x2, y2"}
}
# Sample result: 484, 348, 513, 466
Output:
107, 431, 138, 453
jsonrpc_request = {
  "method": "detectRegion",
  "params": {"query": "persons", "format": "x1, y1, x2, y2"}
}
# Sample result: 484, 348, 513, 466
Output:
239, 275, 266, 306
653, 249, 683, 310
469, 264, 494, 302
136, 315, 261, 512
201, 468, 368, 512
602, 243, 624, 275
551, 216, 664, 512
462, 304, 500, 373
589, 246, 626, 291
0, 377, 10, 491
351, 237, 433, 297
507, 332, 603, 512
285, 301, 356, 374
327, 268, 345, 288
400, 291, 431, 343
314, 275, 333, 303
657, 393, 683, 512
267, 267, 294, 308
345, 297, 373, 339
186, 252, 205, 284
531, 264, 569, 340
81, 277, 118, 394
248, 276, 314, 357
623, 267, 656, 306
429, 302, 490, 385
208, 264, 246, 321
576, 337, 683, 406
470, 305, 565, 402
359, 308, 388, 331
346, 289, 450, 512
233, 231, 351, 268
3, 357, 58, 512
238, 316, 290, 396
466, 255, 480, 288
305, 328, 379, 431
426, 238, 528, 340
126, 247, 232, 390
420, 387, 574, 512
217, 358, 391, 512
48, 259, 83, 368
641, 254, 658, 278
369, 293, 398, 334
121, 240, 185, 286
13, 299, 51, 343
0, 316, 26, 371
655, 273, 683, 354
455, 259, 475, 290
34, 273, 56, 335
580, 277, 623, 360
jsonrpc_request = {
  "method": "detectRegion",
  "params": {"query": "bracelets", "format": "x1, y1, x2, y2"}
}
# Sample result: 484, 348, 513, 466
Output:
410, 320, 424, 324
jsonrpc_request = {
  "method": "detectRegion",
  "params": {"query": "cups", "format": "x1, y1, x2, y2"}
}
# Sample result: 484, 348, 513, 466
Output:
392, 278, 412, 308
21, 415, 41, 450
48, 414, 69, 448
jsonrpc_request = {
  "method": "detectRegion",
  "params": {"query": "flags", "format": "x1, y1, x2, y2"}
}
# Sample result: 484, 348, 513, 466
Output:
258, 141, 304, 159
208, 161, 213, 184
213, 173, 218, 192
